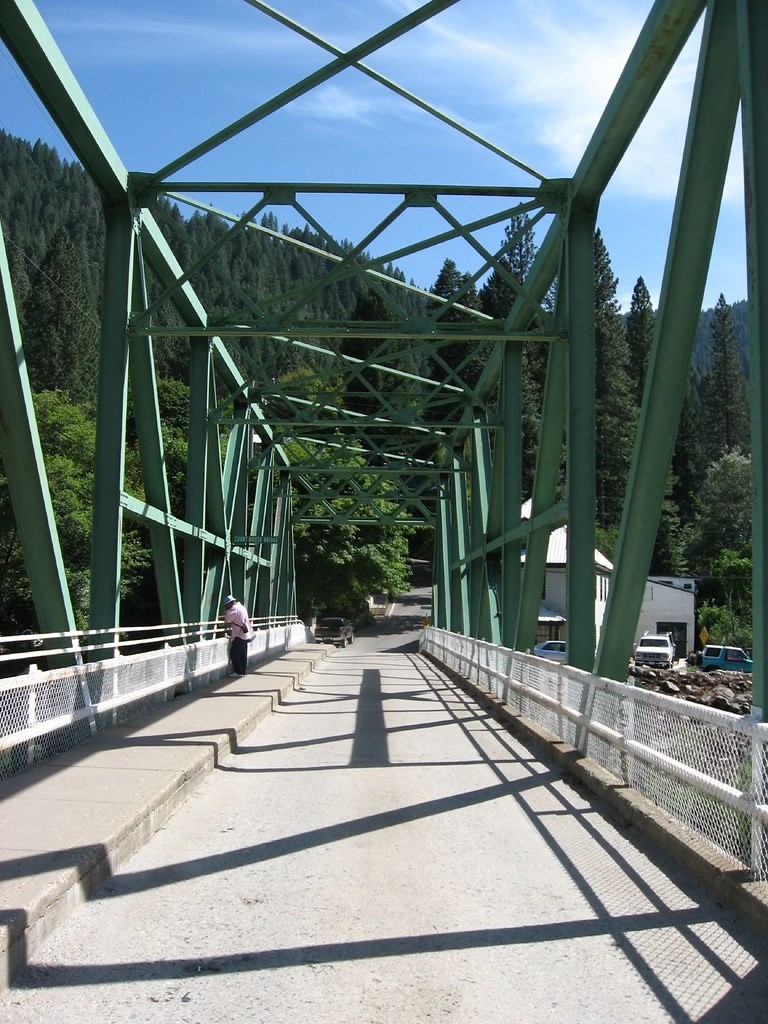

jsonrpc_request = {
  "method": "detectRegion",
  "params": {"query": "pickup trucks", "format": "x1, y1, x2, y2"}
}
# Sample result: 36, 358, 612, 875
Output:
315, 618, 354, 648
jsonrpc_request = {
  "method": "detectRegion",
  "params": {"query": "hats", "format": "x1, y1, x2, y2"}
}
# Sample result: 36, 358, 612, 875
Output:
223, 595, 236, 605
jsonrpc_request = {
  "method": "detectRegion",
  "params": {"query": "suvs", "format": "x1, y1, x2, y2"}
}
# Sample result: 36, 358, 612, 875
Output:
696, 644, 753, 674
633, 631, 677, 669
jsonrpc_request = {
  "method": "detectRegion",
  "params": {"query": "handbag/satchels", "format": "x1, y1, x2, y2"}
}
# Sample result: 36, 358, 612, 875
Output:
239, 622, 256, 642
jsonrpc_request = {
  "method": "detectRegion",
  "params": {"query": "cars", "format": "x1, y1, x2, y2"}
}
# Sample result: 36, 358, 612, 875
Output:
533, 640, 566, 661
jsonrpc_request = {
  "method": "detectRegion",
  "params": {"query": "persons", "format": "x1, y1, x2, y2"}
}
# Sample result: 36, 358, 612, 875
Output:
223, 595, 251, 678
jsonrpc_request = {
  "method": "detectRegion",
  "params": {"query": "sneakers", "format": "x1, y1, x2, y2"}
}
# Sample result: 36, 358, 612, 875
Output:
228, 672, 245, 678
